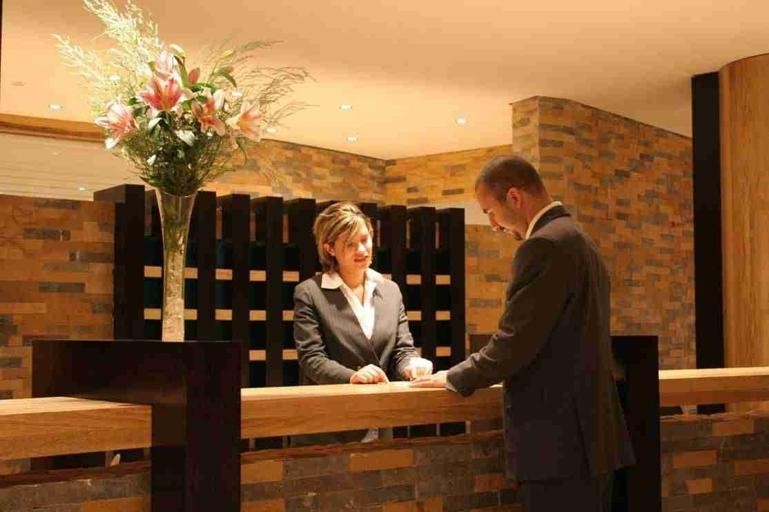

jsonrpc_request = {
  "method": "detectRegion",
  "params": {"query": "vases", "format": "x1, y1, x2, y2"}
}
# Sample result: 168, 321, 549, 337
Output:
152, 187, 197, 342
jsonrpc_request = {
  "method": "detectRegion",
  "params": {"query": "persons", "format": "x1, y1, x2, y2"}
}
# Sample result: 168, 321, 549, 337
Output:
410, 154, 638, 512
290, 202, 434, 448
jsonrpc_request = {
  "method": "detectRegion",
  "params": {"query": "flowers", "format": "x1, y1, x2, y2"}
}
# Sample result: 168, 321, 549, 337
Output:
48, 1, 319, 315
